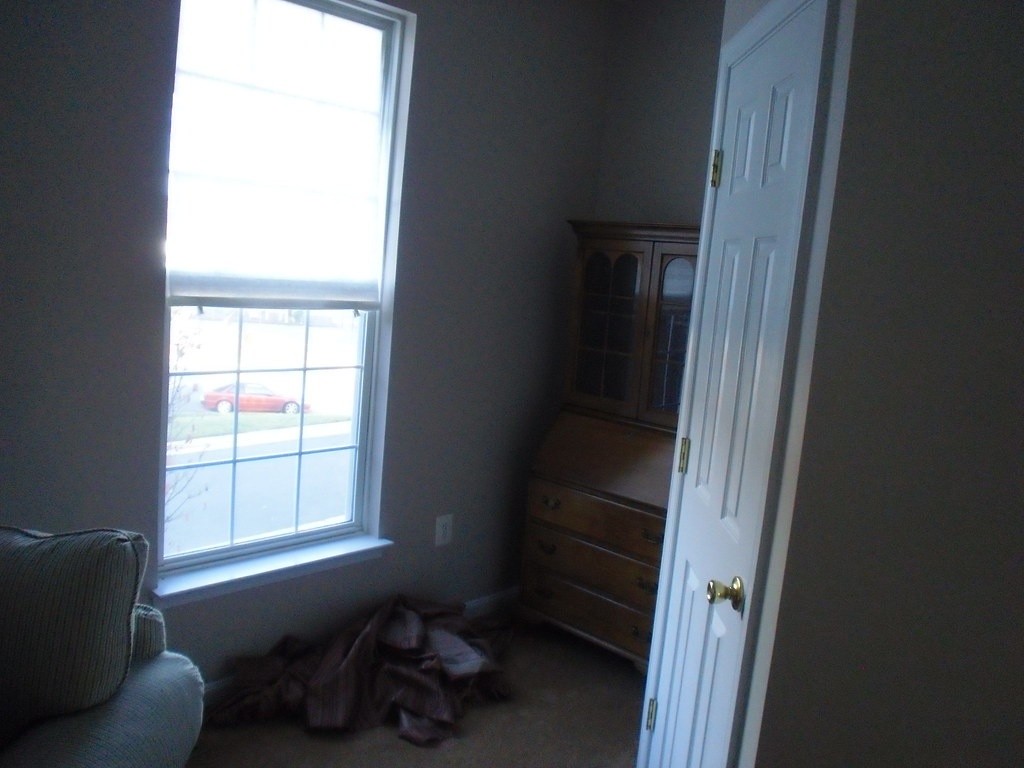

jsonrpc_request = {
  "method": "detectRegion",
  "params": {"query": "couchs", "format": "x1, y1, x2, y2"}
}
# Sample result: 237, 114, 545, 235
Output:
0, 602, 206, 768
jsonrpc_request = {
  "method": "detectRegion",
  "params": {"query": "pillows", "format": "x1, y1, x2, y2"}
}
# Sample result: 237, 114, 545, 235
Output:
0, 524, 150, 735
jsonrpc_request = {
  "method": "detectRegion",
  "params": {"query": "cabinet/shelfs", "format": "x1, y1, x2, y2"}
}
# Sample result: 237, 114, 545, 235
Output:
521, 212, 704, 673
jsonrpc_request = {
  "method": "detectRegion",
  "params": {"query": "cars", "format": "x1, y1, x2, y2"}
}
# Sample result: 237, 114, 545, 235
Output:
201, 381, 310, 414
